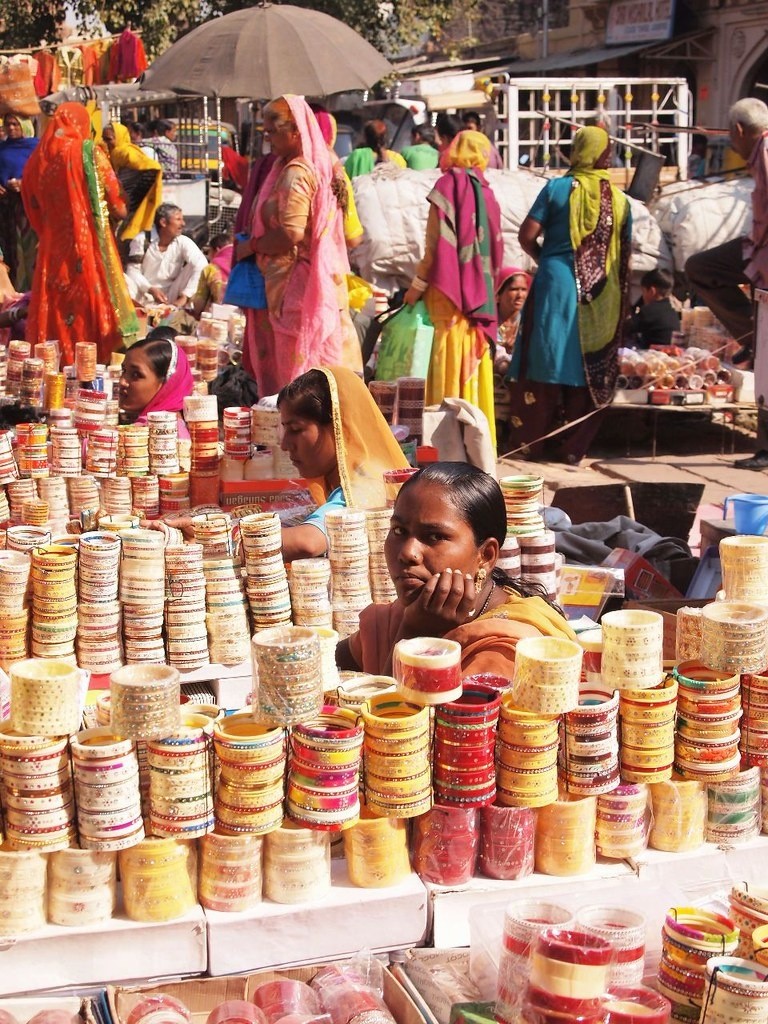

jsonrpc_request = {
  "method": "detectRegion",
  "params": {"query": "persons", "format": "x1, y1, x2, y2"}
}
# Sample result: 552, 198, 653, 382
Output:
186, 95, 366, 408
336, 461, 577, 682
685, 98, 768, 470
119, 326, 194, 440
348, 111, 680, 468
0, 104, 209, 372
140, 369, 412, 560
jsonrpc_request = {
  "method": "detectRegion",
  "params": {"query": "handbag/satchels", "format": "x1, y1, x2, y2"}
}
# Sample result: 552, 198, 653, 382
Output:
347, 300, 408, 367
222, 233, 267, 309
374, 299, 436, 381
209, 367, 259, 421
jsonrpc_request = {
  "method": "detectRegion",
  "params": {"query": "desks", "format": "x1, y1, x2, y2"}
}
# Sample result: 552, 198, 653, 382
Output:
607, 402, 758, 458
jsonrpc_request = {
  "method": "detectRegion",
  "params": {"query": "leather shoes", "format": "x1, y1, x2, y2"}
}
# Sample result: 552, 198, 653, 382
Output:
735, 449, 768, 471
731, 347, 753, 365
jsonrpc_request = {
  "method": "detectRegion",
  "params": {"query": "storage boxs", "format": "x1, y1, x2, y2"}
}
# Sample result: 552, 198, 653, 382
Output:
0, 832, 768, 1024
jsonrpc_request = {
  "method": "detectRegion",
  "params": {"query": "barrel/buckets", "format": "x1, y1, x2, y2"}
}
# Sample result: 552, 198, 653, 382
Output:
724, 494, 768, 536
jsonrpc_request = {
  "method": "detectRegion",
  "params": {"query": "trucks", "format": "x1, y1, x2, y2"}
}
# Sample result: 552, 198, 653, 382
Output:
154, 118, 238, 189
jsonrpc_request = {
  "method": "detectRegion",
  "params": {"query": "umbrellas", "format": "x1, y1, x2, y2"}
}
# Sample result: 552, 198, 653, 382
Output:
141, 2, 394, 101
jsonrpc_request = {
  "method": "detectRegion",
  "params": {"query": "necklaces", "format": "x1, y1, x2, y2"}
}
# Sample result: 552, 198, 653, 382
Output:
478, 580, 496, 614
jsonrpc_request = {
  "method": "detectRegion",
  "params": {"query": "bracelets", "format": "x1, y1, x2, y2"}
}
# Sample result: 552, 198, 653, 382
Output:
412, 275, 429, 292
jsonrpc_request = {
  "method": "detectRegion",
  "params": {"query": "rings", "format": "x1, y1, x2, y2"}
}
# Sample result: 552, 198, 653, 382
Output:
468, 609, 475, 618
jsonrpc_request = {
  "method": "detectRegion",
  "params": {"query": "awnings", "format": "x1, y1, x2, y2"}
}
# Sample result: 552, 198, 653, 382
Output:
500, 29, 717, 76
395, 54, 510, 74
